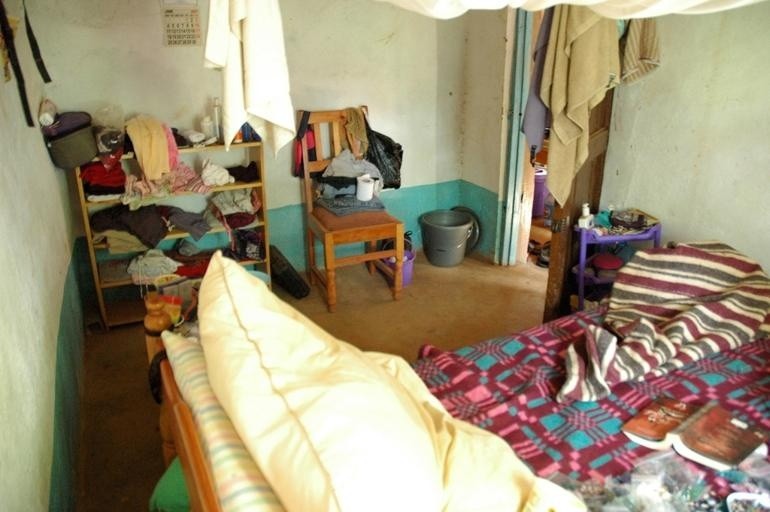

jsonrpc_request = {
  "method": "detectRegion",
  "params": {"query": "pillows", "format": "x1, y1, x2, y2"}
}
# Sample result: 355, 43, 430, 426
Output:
160, 330, 284, 512
197, 250, 443, 512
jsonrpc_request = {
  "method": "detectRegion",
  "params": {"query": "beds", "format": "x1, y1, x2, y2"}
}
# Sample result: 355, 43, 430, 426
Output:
139, 299, 769, 512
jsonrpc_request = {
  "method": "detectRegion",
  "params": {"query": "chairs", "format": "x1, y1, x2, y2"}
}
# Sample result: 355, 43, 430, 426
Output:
295, 106, 405, 313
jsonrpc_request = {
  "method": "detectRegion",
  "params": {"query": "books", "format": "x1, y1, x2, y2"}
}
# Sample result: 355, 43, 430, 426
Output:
619, 397, 766, 473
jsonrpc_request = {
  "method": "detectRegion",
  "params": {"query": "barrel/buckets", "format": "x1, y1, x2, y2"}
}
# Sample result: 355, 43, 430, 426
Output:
382, 239, 416, 286
419, 210, 474, 267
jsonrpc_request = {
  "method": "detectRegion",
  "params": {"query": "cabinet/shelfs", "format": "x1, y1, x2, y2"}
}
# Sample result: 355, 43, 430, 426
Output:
74, 141, 273, 331
571, 220, 662, 312
